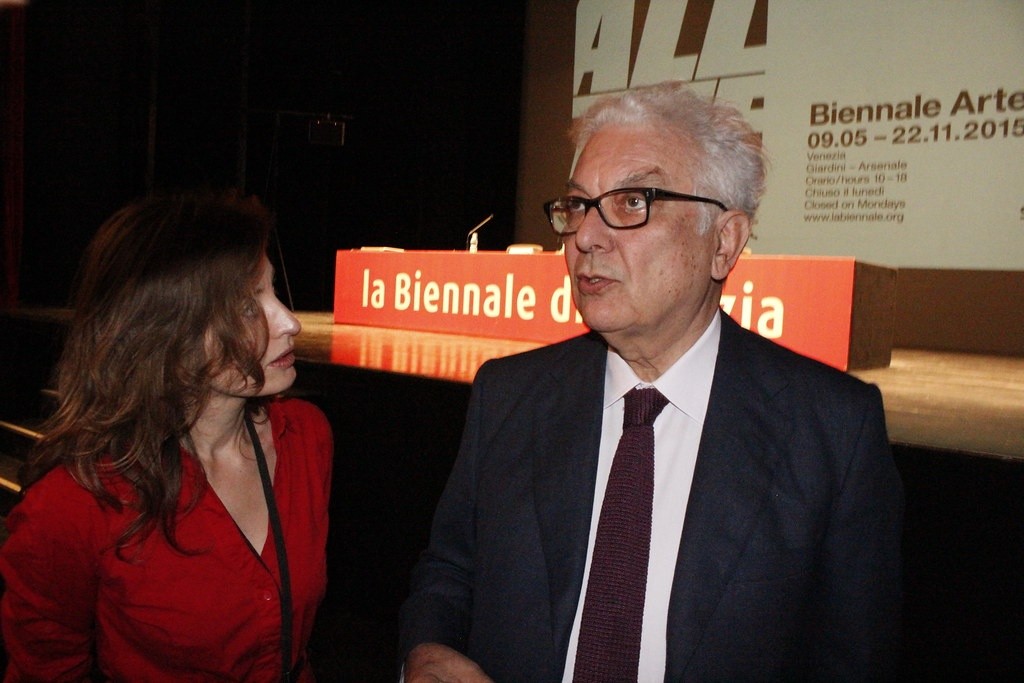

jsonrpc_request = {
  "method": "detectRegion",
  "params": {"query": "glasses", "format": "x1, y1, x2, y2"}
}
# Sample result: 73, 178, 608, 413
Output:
543, 187, 726, 237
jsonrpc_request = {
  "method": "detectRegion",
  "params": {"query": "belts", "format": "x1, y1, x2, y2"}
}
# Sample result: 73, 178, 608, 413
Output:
279, 648, 309, 683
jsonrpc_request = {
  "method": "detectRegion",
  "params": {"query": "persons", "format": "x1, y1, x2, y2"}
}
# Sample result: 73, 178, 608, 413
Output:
394, 78, 908, 683
0, 187, 332, 682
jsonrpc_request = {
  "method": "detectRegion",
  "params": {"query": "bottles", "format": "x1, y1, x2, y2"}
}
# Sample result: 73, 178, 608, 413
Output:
470, 233, 478, 253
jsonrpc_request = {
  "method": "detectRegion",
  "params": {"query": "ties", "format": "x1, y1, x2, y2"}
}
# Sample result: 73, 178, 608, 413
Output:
572, 387, 671, 683
